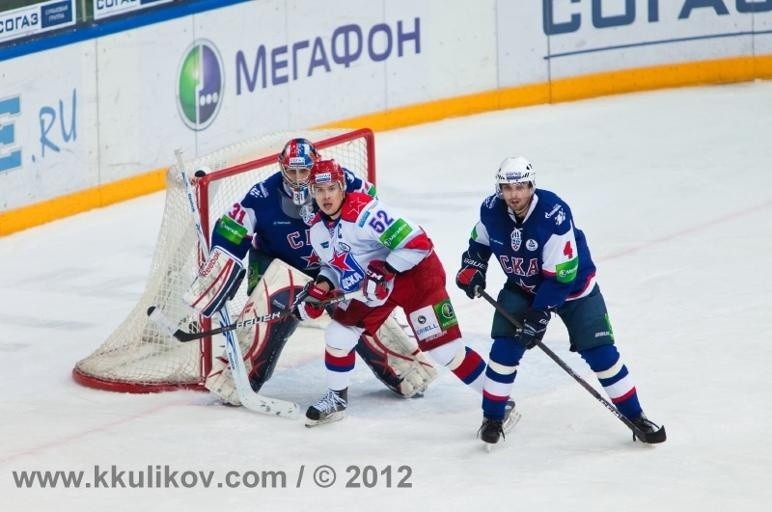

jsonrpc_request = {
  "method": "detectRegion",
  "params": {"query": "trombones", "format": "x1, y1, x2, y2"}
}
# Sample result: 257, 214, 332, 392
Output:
478, 290, 667, 443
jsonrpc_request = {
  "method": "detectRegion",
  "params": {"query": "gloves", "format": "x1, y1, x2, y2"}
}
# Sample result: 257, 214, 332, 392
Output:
515, 311, 551, 349
456, 257, 487, 298
363, 260, 394, 301
290, 282, 325, 321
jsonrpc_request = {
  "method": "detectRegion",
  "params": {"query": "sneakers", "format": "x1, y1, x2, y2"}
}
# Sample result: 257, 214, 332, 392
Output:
477, 397, 516, 443
306, 386, 348, 420
633, 412, 659, 443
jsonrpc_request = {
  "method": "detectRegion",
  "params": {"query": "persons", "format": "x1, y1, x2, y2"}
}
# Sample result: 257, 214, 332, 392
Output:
181, 137, 438, 406
455, 153, 659, 444
289, 158, 520, 435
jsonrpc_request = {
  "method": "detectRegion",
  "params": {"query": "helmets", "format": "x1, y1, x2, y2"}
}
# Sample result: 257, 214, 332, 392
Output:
277, 139, 321, 204
495, 156, 536, 200
307, 159, 347, 198
